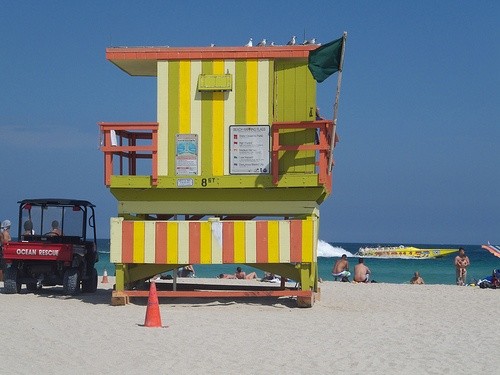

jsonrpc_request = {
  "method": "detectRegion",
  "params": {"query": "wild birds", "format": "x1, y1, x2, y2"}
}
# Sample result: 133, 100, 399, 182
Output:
210, 43, 217, 47
269, 40, 275, 46
244, 37, 253, 47
256, 38, 268, 47
299, 37, 317, 45
286, 34, 297, 45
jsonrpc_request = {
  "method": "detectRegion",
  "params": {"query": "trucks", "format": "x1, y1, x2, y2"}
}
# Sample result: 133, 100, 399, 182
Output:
3, 198, 99, 297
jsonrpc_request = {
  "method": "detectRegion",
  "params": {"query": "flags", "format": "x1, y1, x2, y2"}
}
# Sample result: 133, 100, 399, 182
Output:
308, 36, 346, 84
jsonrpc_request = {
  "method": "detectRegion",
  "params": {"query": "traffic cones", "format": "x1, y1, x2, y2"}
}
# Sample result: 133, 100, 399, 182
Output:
138, 282, 169, 329
101, 269, 109, 283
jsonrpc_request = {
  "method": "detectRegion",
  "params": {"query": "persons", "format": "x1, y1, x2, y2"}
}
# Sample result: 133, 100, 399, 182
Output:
219, 267, 258, 279
48, 220, 62, 237
354, 258, 371, 283
24, 221, 36, 235
182, 265, 195, 278
332, 254, 351, 281
455, 248, 470, 286
0, 220, 12, 282
410, 272, 424, 285
458, 254, 467, 277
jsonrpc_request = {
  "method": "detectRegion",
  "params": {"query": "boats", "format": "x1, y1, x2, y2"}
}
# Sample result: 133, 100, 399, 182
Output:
353, 244, 460, 259
481, 241, 500, 259
